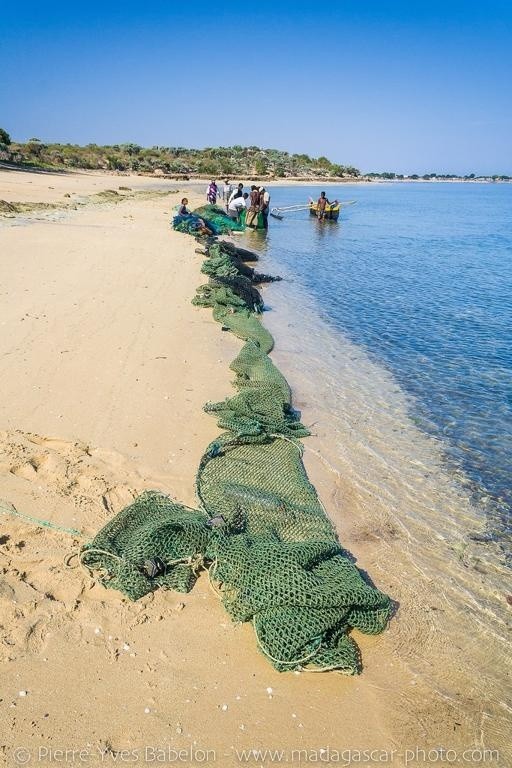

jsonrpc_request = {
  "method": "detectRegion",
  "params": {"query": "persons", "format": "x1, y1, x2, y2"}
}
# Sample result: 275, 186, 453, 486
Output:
205, 179, 222, 204
178, 197, 189, 216
256, 186, 270, 229
245, 184, 260, 232
222, 178, 233, 204
317, 190, 337, 220
228, 182, 243, 204
227, 193, 249, 225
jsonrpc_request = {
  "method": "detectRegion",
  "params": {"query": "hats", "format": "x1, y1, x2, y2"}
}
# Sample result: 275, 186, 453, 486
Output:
259, 187, 263, 192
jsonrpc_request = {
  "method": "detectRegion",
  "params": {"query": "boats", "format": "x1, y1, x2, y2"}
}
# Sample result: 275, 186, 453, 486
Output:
308, 202, 340, 222
